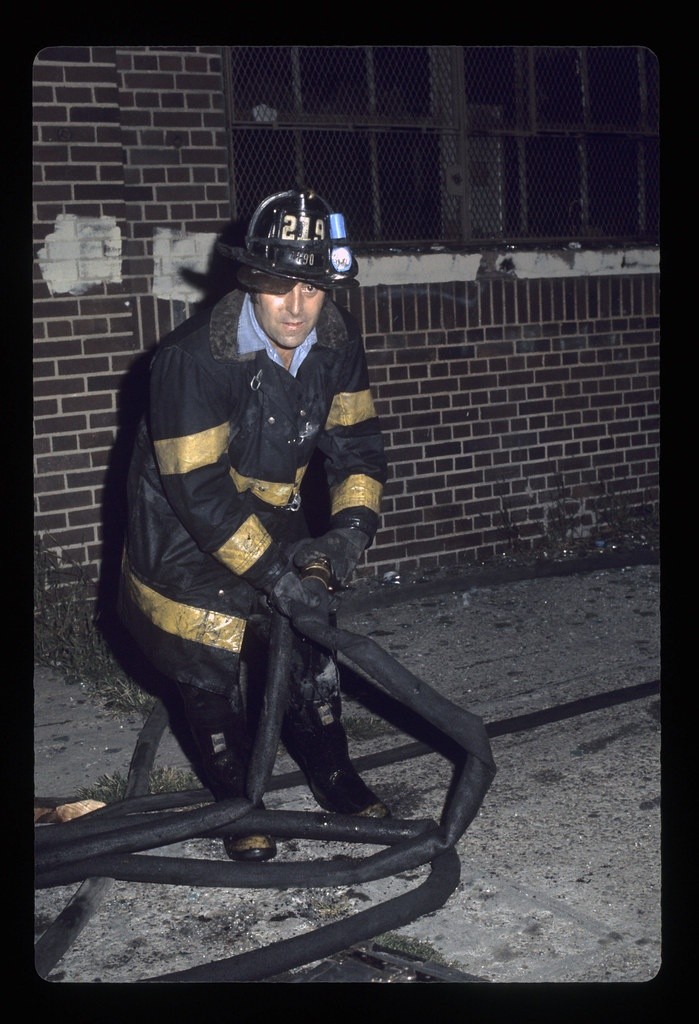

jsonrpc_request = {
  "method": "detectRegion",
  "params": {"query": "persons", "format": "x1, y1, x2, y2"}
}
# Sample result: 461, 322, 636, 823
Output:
115, 187, 392, 866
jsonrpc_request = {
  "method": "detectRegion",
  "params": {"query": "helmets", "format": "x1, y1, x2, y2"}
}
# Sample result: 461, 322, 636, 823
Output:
217, 188, 359, 294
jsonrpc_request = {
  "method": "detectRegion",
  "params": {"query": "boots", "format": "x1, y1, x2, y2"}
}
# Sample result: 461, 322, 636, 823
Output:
293, 718, 391, 820
193, 741, 276, 861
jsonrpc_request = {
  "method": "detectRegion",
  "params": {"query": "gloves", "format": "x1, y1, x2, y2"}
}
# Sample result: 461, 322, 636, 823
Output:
264, 570, 341, 616
293, 527, 369, 592
